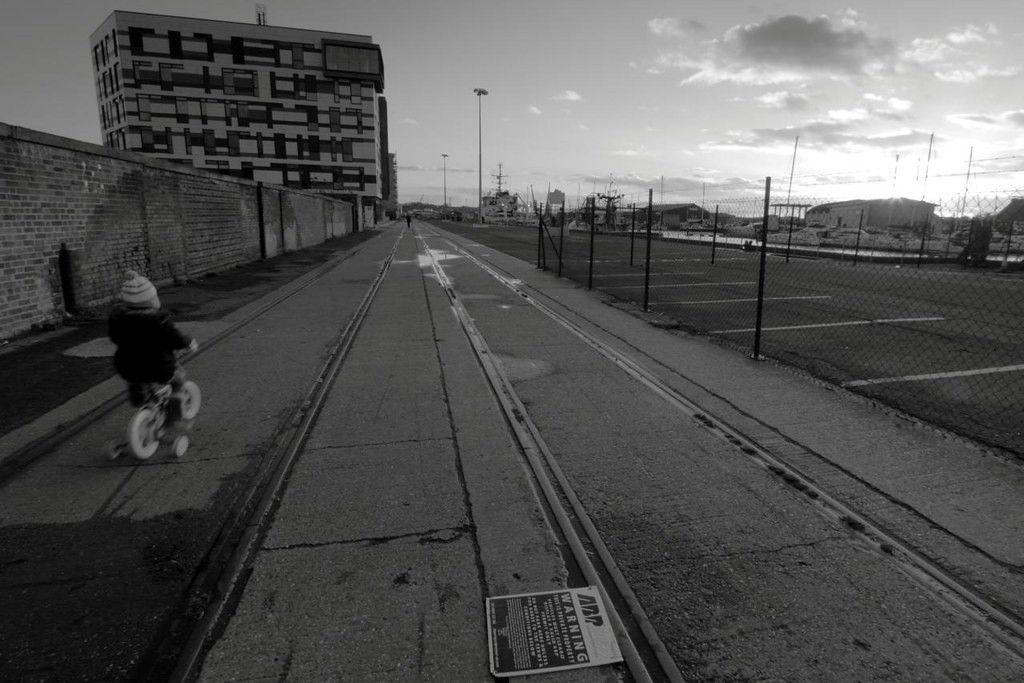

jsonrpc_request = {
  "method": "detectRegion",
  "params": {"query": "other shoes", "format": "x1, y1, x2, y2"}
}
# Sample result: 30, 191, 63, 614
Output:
168, 391, 185, 426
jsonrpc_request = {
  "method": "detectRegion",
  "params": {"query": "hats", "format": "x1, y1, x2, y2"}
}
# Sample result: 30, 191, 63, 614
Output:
121, 269, 161, 312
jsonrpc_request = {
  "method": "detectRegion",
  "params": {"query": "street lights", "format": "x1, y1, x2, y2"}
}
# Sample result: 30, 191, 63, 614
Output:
442, 153, 449, 219
473, 88, 489, 226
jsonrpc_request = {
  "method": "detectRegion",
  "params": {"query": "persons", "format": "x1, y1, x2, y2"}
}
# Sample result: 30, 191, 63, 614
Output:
108, 269, 199, 426
406, 214, 411, 227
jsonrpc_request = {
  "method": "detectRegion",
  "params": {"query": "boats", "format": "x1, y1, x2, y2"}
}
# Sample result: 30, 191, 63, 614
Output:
475, 130, 1023, 258
